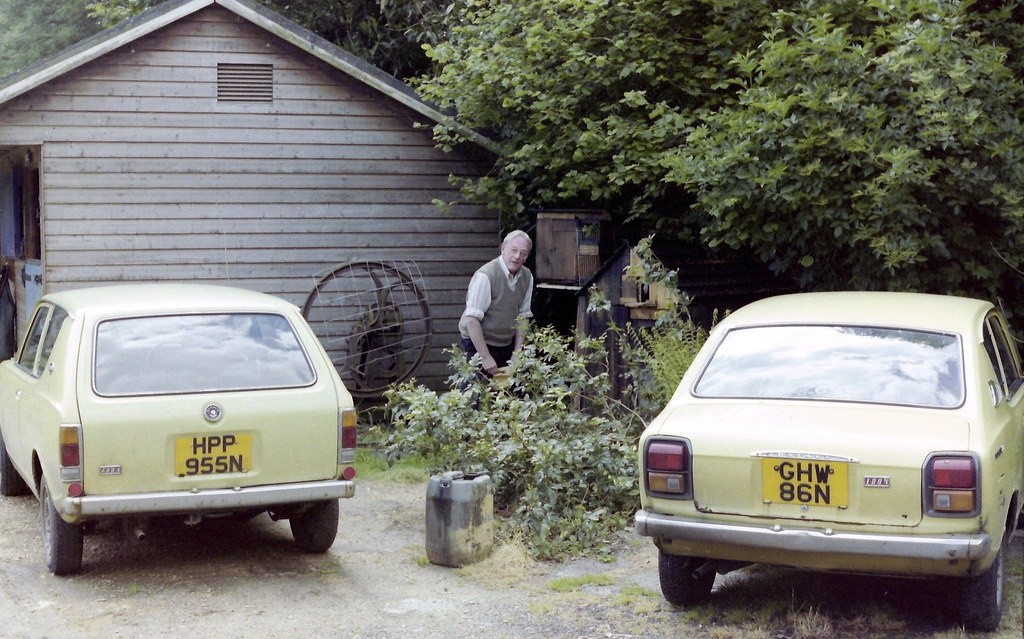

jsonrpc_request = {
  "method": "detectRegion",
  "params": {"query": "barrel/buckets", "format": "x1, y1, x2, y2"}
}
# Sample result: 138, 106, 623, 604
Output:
426, 471, 494, 567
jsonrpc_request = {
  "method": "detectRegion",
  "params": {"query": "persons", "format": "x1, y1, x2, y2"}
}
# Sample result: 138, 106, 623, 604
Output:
457, 229, 535, 384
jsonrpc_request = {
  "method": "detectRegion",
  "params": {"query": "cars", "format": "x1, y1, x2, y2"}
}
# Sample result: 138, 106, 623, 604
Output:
634, 291, 1023, 635
0, 281, 358, 577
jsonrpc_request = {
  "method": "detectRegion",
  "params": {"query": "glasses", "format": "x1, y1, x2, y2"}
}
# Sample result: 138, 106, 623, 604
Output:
505, 242, 529, 258
